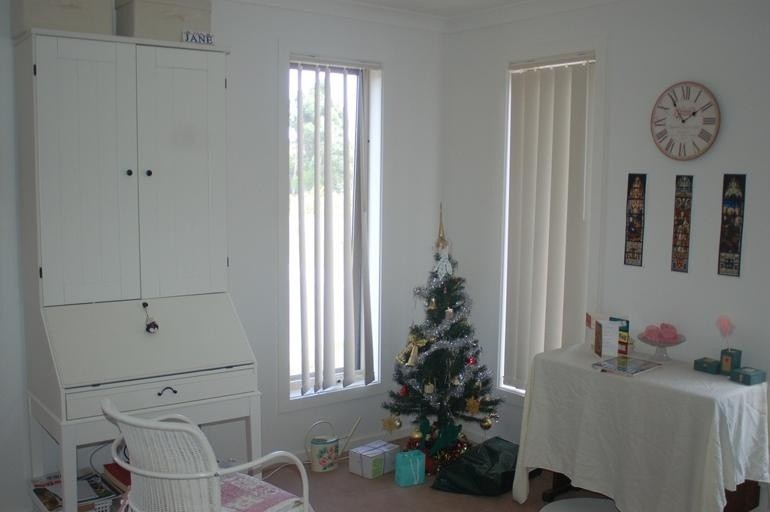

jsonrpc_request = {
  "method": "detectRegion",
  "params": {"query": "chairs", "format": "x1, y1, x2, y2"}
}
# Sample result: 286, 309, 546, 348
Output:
102, 398, 313, 512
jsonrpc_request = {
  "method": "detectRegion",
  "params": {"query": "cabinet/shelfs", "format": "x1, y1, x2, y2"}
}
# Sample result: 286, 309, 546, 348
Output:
13, 29, 263, 512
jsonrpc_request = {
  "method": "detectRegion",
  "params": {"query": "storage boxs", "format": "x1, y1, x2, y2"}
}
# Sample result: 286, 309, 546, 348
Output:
9, 1, 116, 40
348, 440, 400, 480
115, 0, 212, 43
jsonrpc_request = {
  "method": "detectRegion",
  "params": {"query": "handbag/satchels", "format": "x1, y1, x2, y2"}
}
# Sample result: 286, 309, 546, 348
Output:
395, 450, 426, 487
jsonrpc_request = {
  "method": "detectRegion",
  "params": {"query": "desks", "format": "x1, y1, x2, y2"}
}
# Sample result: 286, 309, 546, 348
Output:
511, 342, 770, 512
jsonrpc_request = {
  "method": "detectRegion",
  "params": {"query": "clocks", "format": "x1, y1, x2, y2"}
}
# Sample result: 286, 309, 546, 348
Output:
650, 81, 722, 159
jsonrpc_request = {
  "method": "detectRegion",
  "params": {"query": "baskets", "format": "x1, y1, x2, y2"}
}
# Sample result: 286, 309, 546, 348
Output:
31, 475, 115, 512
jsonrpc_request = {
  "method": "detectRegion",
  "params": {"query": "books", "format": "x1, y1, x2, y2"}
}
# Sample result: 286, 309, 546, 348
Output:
594, 319, 620, 360
593, 356, 662, 376
33, 462, 131, 512
